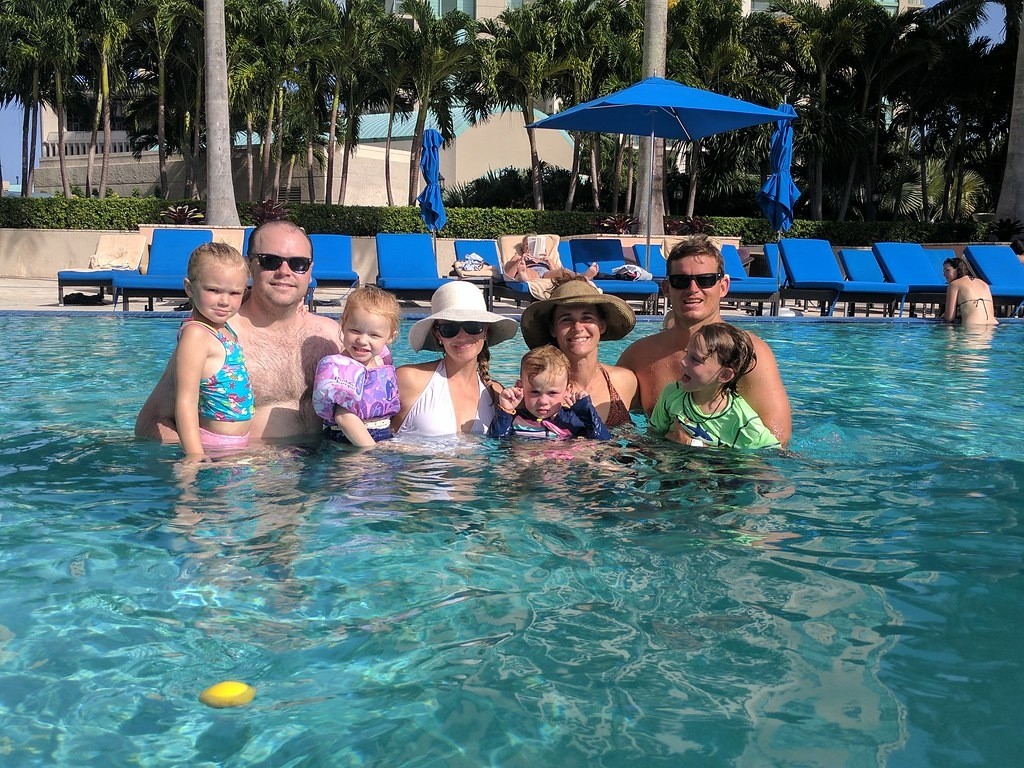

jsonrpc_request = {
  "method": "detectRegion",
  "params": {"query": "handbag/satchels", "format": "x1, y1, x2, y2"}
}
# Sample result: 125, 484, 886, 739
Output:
454, 260, 500, 281
612, 265, 652, 281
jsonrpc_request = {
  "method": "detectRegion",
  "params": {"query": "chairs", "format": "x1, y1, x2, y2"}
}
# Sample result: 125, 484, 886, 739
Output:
56, 227, 1024, 316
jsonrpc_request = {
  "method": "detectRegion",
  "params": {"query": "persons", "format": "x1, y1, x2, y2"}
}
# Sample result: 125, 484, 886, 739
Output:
520, 279, 639, 439
505, 232, 599, 283
614, 234, 793, 453
312, 284, 401, 449
940, 257, 999, 326
484, 345, 610, 441
134, 220, 344, 455
391, 281, 518, 439
177, 244, 254, 466
647, 321, 783, 450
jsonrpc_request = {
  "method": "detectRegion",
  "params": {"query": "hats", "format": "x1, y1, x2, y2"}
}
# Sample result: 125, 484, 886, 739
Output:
520, 280, 636, 350
409, 281, 518, 353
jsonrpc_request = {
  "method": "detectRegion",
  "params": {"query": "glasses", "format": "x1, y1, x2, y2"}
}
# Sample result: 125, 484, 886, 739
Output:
248, 252, 313, 274
667, 273, 725, 290
433, 321, 485, 338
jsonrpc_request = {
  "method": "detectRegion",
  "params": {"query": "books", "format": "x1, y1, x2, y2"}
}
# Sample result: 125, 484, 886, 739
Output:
526, 235, 546, 259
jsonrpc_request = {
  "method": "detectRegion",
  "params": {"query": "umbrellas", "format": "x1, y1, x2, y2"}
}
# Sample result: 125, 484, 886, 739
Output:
415, 129, 448, 263
524, 68, 795, 271
759, 95, 802, 243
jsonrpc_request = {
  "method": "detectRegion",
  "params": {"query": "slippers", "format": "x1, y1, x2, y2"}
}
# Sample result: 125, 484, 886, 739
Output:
174, 302, 192, 311
